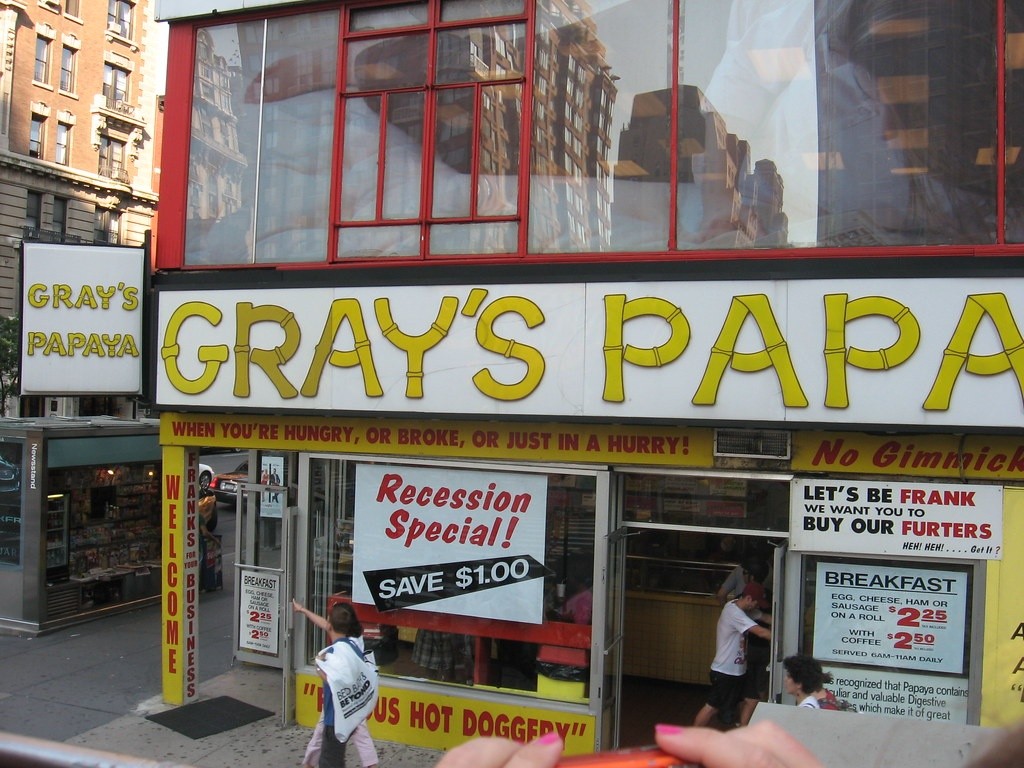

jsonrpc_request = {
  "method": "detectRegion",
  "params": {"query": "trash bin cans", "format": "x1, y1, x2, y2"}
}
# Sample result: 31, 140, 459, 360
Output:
200, 535, 223, 593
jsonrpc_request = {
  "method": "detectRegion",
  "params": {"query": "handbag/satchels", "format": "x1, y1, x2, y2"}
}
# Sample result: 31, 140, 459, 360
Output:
316, 641, 379, 744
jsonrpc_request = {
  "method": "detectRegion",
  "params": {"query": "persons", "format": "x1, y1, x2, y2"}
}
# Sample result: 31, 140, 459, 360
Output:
408, 628, 474, 684
538, 566, 592, 682
434, 721, 820, 768
262, 469, 281, 503
695, 582, 783, 728
716, 537, 772, 699
783, 655, 839, 710
292, 598, 379, 768
199, 513, 221, 594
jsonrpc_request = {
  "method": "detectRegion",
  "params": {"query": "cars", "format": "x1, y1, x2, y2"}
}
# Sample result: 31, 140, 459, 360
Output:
197, 463, 216, 500
211, 459, 261, 506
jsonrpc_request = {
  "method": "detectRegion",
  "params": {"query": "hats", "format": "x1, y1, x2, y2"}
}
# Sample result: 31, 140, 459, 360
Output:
745, 582, 769, 608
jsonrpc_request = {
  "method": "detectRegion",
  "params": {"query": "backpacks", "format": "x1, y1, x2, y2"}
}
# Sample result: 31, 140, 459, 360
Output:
819, 697, 857, 712
